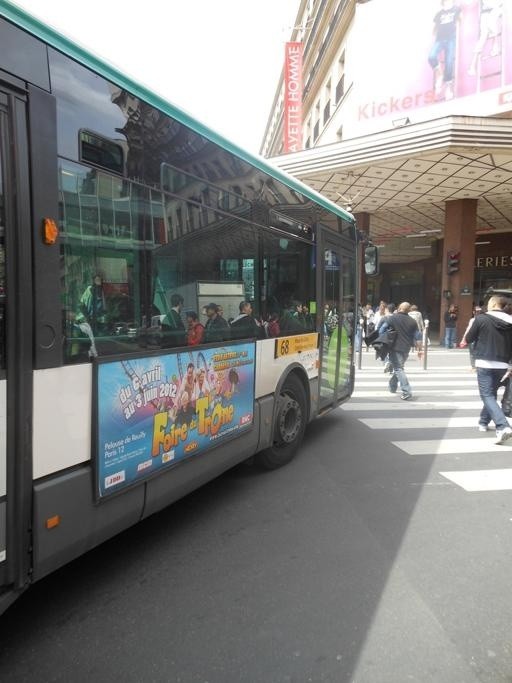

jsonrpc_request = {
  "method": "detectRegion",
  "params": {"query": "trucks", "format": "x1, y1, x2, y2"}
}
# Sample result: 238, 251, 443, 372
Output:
162, 278, 247, 331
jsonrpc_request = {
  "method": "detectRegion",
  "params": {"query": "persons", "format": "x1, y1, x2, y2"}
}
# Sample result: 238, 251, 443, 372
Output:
66, 271, 354, 355
356, 300, 512, 414
173, 362, 196, 414
465, 294, 512, 445
192, 368, 212, 400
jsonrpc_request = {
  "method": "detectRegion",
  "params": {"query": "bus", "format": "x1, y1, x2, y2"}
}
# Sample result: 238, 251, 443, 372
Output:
1, 0, 381, 623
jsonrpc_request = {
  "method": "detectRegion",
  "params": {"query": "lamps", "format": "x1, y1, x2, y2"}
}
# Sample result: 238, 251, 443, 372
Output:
391, 117, 412, 129
443, 290, 453, 300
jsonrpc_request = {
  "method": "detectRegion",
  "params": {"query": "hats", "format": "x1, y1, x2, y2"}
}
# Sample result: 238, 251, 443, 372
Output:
202, 302, 217, 308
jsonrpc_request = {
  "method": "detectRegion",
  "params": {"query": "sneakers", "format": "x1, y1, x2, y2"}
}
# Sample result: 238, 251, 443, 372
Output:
494, 427, 512, 445
479, 423, 489, 431
400, 394, 412, 400
387, 385, 397, 392
